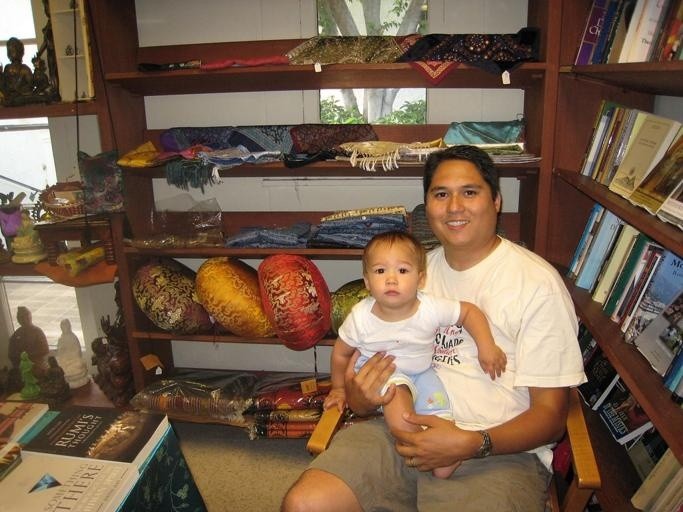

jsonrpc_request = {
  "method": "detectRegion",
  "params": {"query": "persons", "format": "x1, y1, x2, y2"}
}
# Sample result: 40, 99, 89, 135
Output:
16, 350, 39, 397
37, 355, 63, 395
0, 37, 33, 108
317, 230, 506, 477
11, 209, 41, 249
36, 0, 62, 102
282, 146, 587, 510
53, 318, 88, 381
6, 304, 48, 368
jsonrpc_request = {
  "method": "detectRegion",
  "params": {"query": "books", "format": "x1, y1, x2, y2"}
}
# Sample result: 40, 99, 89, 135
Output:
564, 0, 683, 512
0, 398, 172, 511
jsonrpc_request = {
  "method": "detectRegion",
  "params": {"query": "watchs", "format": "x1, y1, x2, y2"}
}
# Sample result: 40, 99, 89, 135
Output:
474, 429, 494, 459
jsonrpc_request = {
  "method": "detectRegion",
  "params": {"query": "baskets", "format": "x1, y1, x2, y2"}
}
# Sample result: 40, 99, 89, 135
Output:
39, 181, 85, 216
